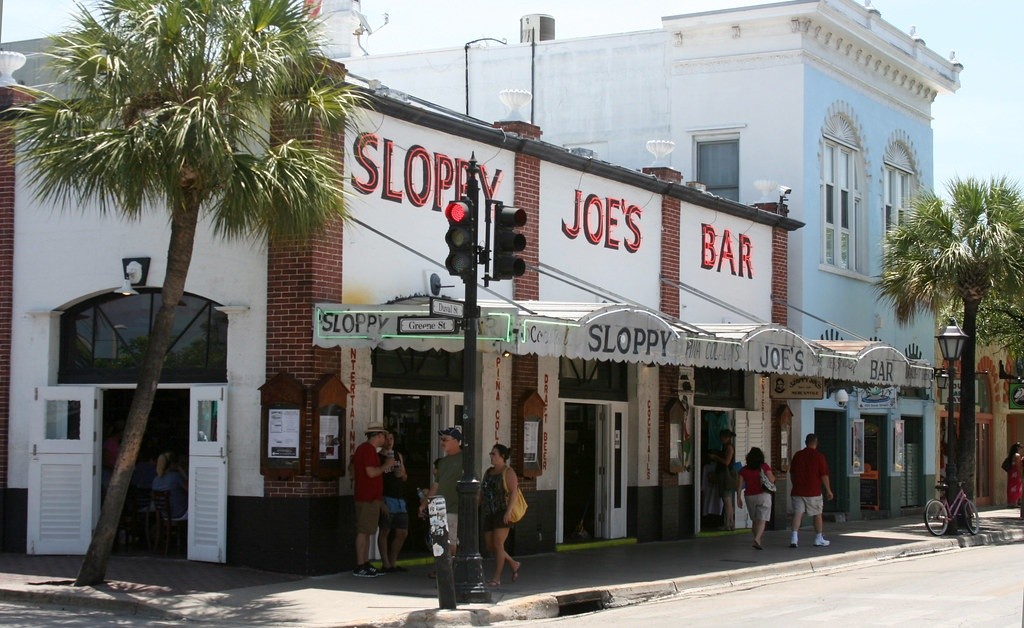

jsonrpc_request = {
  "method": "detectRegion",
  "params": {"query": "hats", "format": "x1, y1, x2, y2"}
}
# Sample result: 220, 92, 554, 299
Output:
438, 427, 462, 440
364, 422, 389, 434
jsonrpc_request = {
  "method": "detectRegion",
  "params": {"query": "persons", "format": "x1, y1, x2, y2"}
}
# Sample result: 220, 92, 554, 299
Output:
1007, 441, 1022, 508
789, 434, 833, 548
377, 428, 410, 572
102, 420, 188, 521
418, 427, 464, 579
736, 447, 775, 550
708, 429, 738, 531
479, 444, 521, 587
353, 422, 395, 577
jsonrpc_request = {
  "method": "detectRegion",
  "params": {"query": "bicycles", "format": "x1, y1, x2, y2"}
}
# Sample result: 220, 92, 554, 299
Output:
923, 479, 979, 536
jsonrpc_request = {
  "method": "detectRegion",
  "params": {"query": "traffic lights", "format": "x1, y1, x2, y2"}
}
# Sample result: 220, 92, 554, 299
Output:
495, 202, 527, 283
444, 201, 473, 276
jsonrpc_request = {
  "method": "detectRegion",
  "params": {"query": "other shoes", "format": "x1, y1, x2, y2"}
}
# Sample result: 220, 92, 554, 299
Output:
717, 526, 735, 530
1012, 505, 1022, 508
393, 565, 409, 572
381, 566, 398, 573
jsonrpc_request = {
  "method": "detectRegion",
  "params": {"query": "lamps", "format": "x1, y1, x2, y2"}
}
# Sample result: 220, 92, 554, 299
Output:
502, 350, 511, 357
764, 372, 770, 378
932, 367, 949, 390
647, 360, 655, 367
114, 257, 151, 295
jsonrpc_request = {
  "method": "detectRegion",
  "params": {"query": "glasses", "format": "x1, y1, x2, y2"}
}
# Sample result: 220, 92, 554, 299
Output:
441, 438, 448, 442
489, 452, 496, 456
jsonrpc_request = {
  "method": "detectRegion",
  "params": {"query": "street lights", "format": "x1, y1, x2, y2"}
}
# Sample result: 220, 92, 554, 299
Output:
933, 317, 970, 532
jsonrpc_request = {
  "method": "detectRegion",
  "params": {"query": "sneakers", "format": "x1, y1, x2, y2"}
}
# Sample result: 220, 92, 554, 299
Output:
813, 538, 831, 547
353, 564, 385, 577
791, 537, 798, 546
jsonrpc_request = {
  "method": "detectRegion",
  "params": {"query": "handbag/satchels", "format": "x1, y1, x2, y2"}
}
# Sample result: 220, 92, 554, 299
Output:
503, 467, 528, 522
734, 462, 746, 489
759, 468, 776, 493
1002, 458, 1011, 471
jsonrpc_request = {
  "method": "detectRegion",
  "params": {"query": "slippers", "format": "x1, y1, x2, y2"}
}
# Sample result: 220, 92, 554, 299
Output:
486, 580, 500, 587
752, 544, 763, 550
512, 562, 521, 581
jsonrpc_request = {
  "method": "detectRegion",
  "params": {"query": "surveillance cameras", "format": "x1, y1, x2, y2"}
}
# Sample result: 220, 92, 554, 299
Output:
834, 389, 848, 406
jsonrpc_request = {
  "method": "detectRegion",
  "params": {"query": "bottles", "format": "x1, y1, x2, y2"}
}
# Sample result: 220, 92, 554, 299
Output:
417, 488, 425, 500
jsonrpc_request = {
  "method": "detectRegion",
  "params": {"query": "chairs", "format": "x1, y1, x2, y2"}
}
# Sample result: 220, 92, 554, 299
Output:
112, 486, 186, 556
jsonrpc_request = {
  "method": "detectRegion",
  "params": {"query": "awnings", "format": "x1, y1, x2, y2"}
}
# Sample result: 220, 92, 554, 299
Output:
313, 291, 935, 388
